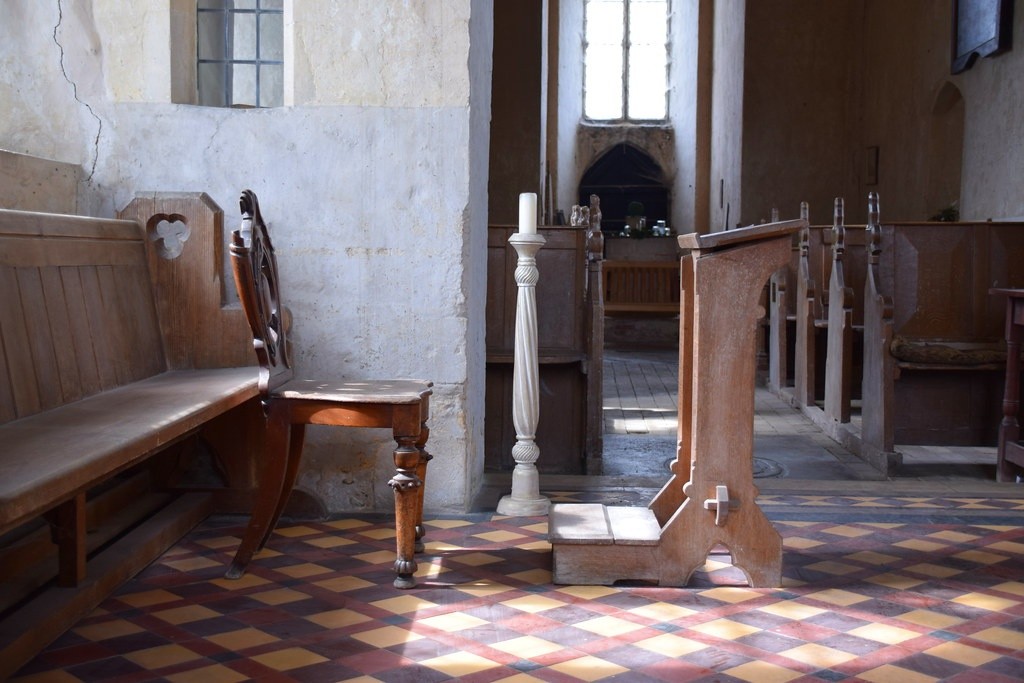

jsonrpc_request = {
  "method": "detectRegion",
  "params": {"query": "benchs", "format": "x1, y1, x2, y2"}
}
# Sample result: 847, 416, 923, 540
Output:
0, 188, 292, 683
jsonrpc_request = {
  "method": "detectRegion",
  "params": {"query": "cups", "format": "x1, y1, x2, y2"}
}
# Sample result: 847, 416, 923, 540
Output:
619, 232, 624, 236
625, 226, 631, 237
665, 228, 671, 236
653, 226, 658, 236
658, 221, 665, 237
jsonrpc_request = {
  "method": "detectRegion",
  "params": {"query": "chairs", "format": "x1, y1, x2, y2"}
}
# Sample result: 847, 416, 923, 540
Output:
225, 187, 436, 589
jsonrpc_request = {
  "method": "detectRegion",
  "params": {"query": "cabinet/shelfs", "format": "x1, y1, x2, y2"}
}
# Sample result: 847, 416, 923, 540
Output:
485, 227, 585, 363
